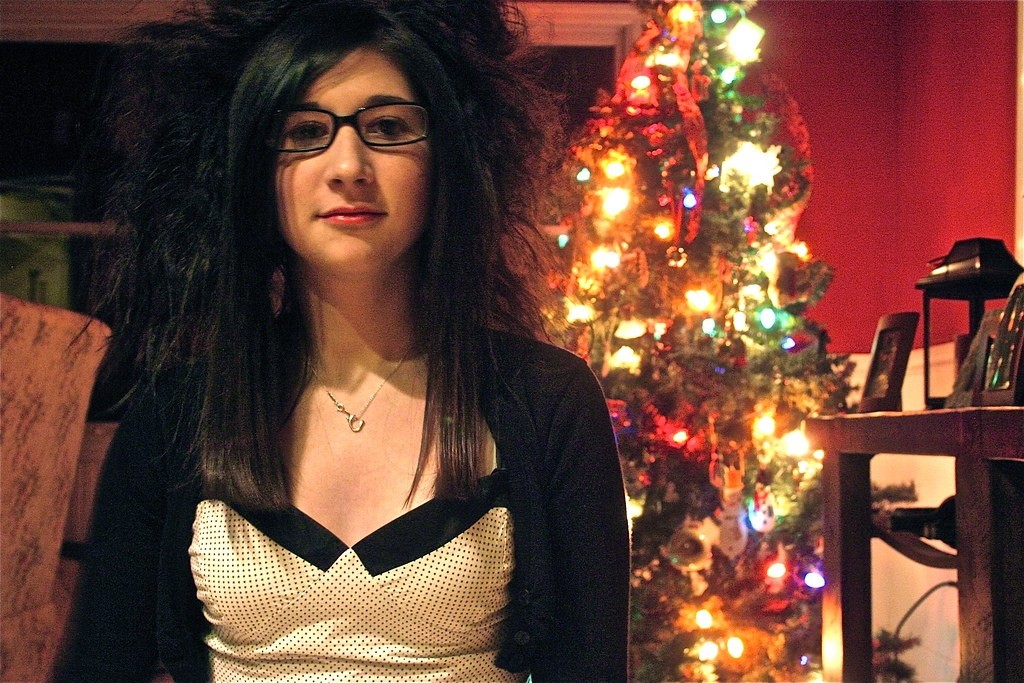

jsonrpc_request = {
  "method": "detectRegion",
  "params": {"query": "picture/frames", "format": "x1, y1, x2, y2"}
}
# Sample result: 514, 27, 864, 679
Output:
856, 312, 920, 414
979, 272, 1024, 406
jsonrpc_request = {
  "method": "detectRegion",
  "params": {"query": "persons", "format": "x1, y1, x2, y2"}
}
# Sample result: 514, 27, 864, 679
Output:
47, 0, 630, 682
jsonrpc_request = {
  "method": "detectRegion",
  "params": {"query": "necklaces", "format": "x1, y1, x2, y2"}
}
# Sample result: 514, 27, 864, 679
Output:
306, 345, 416, 432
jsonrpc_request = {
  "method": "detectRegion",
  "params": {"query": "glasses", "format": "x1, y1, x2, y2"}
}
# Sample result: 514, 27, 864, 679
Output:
265, 100, 431, 153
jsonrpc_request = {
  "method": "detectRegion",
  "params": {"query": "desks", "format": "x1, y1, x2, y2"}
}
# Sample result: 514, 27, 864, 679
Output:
805, 405, 1024, 683
